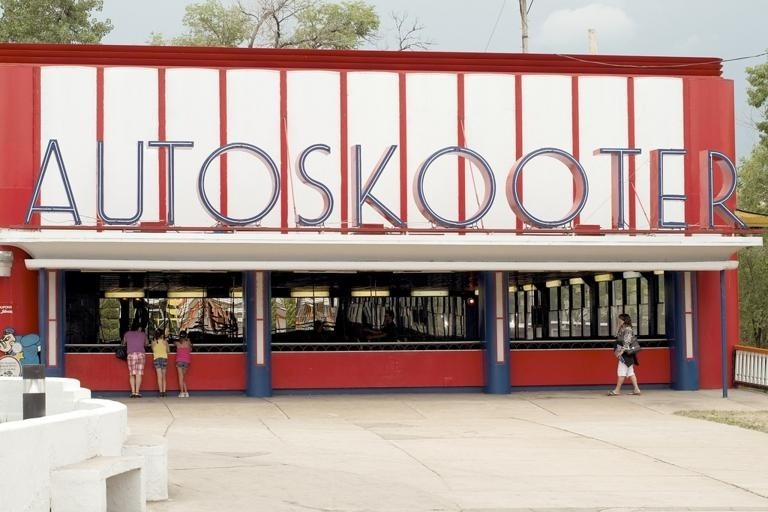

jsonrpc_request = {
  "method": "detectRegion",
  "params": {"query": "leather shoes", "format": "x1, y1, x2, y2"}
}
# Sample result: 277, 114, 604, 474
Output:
129, 393, 142, 398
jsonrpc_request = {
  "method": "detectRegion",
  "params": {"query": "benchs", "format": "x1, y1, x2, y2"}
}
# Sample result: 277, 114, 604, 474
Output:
49, 455, 146, 512
121, 433, 168, 501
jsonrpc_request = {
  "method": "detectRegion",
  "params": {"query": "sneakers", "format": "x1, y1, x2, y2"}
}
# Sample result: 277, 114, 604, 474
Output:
159, 392, 189, 398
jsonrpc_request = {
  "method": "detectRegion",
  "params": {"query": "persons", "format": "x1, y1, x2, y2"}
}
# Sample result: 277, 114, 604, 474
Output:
174, 329, 192, 397
370, 311, 396, 340
149, 329, 169, 398
606, 314, 639, 395
121, 320, 150, 397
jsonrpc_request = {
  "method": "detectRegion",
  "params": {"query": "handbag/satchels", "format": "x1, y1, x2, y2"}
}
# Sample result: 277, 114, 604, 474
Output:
621, 336, 640, 355
115, 342, 126, 360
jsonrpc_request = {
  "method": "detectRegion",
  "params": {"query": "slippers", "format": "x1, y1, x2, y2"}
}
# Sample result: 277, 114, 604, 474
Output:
609, 391, 639, 396
